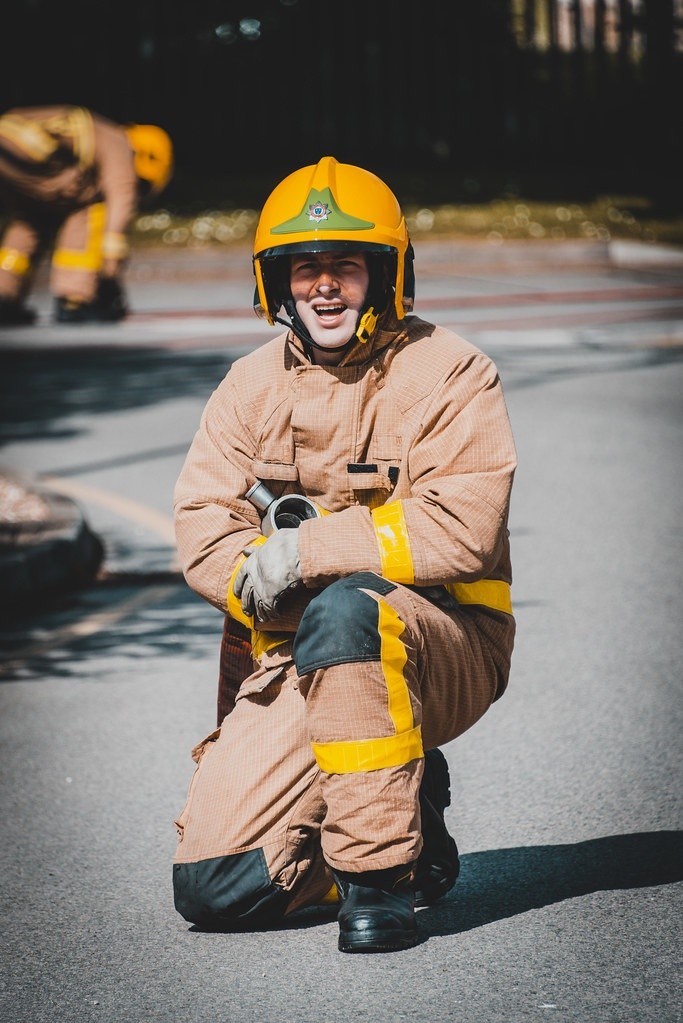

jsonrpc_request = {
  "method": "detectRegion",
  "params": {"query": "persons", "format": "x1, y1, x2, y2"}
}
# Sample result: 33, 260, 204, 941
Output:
171, 155, 517, 952
0, 104, 174, 324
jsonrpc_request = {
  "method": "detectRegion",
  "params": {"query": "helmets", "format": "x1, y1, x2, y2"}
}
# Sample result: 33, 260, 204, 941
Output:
252, 155, 416, 326
124, 123, 171, 196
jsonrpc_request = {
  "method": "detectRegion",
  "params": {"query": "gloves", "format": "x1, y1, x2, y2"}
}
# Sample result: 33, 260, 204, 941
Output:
233, 527, 303, 622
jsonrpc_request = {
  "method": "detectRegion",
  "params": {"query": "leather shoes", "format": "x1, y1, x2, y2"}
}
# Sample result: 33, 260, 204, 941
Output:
421, 750, 461, 903
331, 862, 420, 951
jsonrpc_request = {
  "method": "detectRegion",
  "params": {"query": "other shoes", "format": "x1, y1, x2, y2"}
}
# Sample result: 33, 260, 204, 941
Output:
60, 302, 124, 321
0, 303, 33, 326
